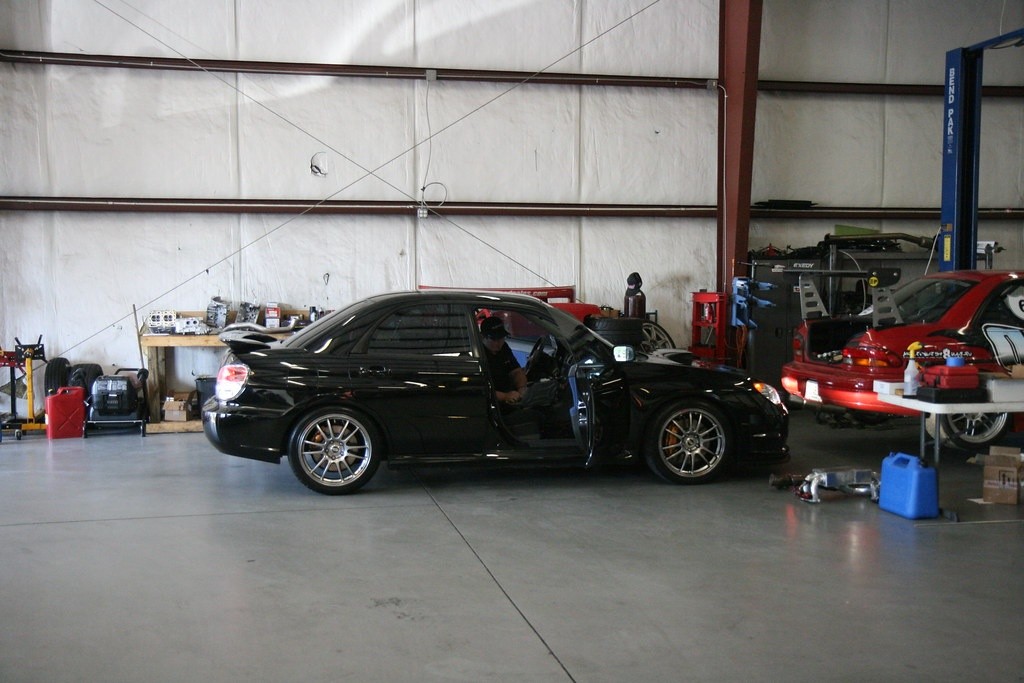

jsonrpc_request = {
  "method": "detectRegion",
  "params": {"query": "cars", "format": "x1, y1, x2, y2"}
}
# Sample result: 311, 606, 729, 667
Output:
770, 264, 1024, 453
199, 286, 790, 497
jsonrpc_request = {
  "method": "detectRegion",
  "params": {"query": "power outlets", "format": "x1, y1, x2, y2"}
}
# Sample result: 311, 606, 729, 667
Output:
264, 307, 282, 319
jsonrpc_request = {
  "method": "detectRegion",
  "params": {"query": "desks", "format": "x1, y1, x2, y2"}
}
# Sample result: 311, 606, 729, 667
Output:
138, 310, 310, 434
878, 393, 1023, 522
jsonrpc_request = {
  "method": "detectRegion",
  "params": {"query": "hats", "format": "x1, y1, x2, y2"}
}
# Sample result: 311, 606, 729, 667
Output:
480, 317, 510, 339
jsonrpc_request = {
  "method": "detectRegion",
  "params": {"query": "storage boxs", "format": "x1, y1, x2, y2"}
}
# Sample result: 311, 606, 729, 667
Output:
967, 446, 1023, 504
873, 379, 922, 394
263, 318, 281, 328
922, 364, 980, 389
162, 390, 198, 421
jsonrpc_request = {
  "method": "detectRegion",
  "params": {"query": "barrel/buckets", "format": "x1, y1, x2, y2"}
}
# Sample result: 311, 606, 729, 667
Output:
878, 450, 939, 520
44, 386, 85, 440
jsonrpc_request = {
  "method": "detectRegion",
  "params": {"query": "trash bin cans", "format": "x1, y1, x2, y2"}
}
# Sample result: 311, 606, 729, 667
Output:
195, 376, 217, 419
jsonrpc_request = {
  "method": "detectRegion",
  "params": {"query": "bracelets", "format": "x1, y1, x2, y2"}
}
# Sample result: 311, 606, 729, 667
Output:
517, 386, 526, 390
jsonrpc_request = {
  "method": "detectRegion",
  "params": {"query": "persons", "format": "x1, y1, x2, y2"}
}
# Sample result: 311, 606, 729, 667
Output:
459, 317, 528, 415
